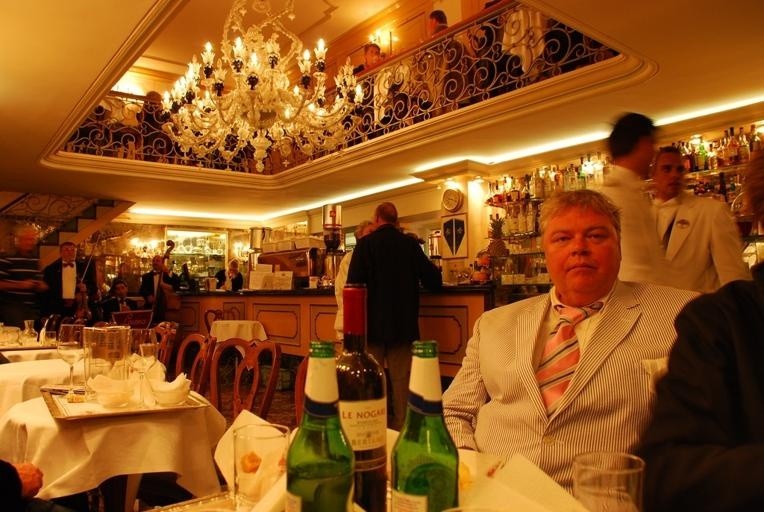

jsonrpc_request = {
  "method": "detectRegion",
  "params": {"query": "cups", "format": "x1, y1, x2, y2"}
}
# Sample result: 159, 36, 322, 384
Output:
569, 449, 647, 512
233, 423, 290, 512
123, 328, 158, 410
43, 330, 56, 345
57, 324, 85, 404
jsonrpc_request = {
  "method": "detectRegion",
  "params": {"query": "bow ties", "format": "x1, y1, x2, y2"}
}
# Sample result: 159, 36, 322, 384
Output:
63, 263, 74, 267
155, 271, 161, 276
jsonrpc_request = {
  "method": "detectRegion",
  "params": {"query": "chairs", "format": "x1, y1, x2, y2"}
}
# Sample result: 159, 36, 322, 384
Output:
156, 328, 177, 367
59, 324, 85, 348
131, 329, 151, 353
44, 313, 60, 339
94, 320, 116, 327
61, 316, 83, 325
210, 338, 281, 427
154, 322, 179, 337
174, 334, 217, 393
294, 353, 310, 425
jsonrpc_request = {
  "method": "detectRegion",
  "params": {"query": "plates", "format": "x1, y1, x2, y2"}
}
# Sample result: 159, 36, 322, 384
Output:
39, 387, 211, 421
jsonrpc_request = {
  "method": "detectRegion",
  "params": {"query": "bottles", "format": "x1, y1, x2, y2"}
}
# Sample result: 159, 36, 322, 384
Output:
668, 140, 696, 172
738, 128, 750, 163
707, 144, 718, 170
695, 136, 706, 170
750, 126, 760, 160
390, 341, 459, 512
333, 283, 387, 512
490, 168, 552, 199
285, 341, 354, 512
489, 205, 543, 235
689, 172, 741, 204
727, 128, 739, 165
711, 131, 731, 165
551, 152, 612, 194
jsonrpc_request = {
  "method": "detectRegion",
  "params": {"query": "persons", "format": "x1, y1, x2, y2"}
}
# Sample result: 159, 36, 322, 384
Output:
427, 10, 476, 87
102, 278, 136, 320
45, 242, 94, 316
347, 202, 442, 429
438, 192, 703, 499
141, 257, 179, 308
353, 44, 381, 74
214, 259, 243, 291
477, 0, 526, 81
336, 220, 373, 351
141, 92, 162, 146
636, 133, 764, 512
645, 147, 749, 292
600, 113, 677, 283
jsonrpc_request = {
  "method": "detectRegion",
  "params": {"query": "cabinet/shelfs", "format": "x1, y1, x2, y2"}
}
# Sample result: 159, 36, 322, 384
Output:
163, 225, 230, 288
229, 228, 251, 287
483, 119, 764, 283
104, 221, 162, 288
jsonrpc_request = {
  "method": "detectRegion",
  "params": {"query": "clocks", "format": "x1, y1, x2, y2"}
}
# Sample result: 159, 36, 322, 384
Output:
442, 188, 459, 211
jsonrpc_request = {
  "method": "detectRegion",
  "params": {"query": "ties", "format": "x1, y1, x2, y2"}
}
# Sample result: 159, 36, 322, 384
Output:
532, 305, 599, 417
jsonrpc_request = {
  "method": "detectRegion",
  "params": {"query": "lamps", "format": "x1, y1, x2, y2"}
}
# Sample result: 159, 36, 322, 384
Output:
160, 0, 364, 172
